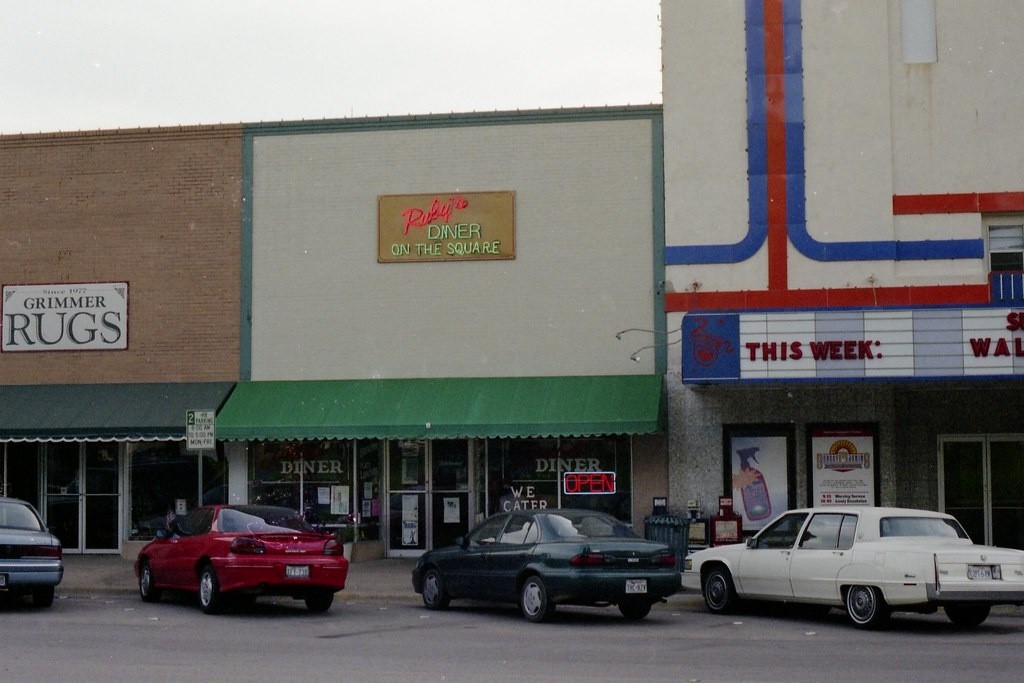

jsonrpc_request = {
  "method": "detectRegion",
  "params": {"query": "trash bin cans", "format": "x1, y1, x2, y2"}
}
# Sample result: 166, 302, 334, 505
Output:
645, 514, 686, 572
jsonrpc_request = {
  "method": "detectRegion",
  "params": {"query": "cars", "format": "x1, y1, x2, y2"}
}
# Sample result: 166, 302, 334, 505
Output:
680, 503, 1024, 629
134, 503, 350, 612
412, 509, 682, 624
1, 497, 65, 608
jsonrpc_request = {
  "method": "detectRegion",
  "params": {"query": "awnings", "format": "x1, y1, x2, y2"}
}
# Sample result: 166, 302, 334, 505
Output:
206, 375, 669, 441
0, 382, 237, 441
